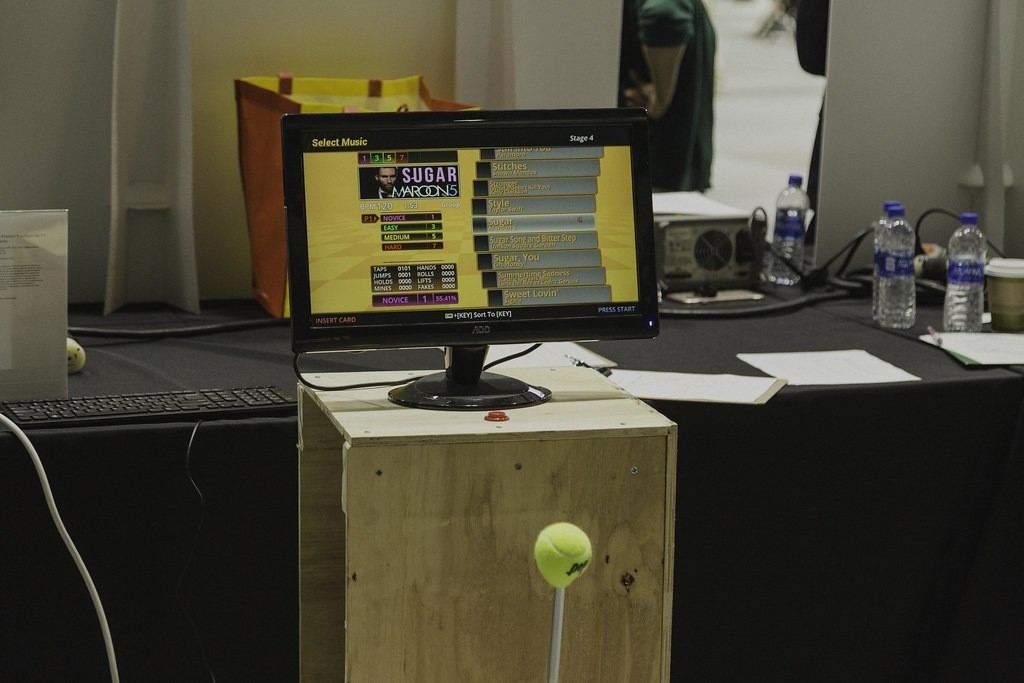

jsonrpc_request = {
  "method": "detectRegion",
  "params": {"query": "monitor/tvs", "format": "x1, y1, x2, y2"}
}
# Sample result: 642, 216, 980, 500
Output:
280, 106, 660, 411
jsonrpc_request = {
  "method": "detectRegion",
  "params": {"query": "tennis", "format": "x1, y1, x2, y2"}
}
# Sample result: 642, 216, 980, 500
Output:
533, 521, 594, 590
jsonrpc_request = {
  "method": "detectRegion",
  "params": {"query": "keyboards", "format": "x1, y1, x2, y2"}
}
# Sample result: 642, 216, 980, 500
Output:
0, 384, 298, 428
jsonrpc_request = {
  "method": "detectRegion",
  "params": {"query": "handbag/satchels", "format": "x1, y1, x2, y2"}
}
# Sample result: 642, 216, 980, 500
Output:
233, 74, 484, 325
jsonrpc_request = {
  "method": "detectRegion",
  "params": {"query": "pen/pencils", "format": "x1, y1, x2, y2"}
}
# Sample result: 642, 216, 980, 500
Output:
927, 326, 943, 345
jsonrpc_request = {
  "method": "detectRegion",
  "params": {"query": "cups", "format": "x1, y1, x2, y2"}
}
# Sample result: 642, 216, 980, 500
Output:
984, 257, 1024, 332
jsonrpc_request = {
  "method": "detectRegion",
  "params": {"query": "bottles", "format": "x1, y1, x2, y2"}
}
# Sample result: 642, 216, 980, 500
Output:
768, 175, 810, 286
941, 212, 987, 333
872, 202, 916, 329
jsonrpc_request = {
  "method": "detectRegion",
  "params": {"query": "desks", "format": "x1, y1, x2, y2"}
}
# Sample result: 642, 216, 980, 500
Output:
0, 283, 1024, 683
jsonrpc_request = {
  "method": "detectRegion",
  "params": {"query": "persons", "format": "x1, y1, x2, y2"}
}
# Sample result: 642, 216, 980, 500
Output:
619, 0, 717, 195
373, 167, 399, 199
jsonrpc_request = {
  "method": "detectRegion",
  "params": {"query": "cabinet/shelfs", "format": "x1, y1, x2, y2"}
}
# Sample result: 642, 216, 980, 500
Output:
298, 369, 677, 683
